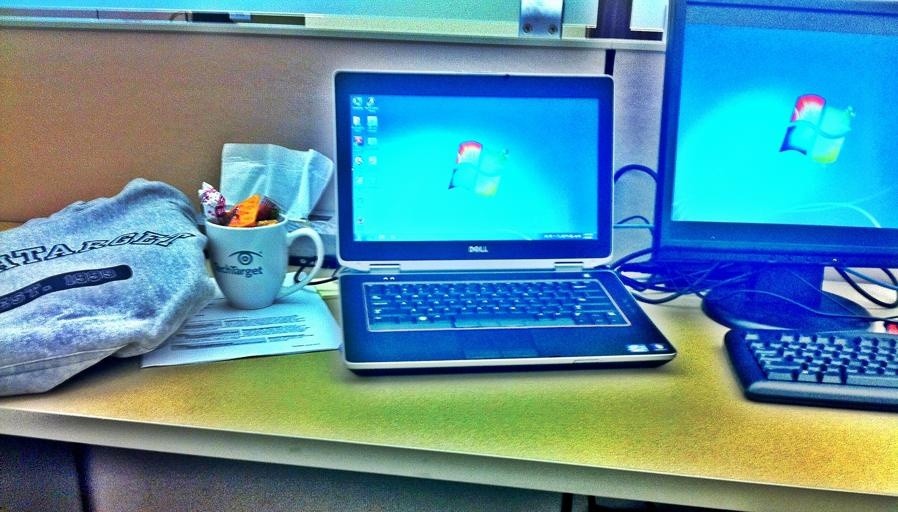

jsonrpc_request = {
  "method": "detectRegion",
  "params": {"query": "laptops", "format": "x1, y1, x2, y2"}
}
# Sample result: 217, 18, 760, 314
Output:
332, 67, 678, 380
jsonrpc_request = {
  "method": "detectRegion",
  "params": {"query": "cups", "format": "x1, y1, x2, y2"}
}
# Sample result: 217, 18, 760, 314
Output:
205, 215, 323, 308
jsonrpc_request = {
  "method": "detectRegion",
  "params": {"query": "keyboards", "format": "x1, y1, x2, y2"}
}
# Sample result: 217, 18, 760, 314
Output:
721, 324, 898, 409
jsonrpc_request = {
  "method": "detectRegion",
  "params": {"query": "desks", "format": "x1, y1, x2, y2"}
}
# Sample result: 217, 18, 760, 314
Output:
0, 279, 898, 509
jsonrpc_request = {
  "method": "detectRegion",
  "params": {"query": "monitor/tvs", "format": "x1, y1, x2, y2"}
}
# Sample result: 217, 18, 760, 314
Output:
652, 0, 898, 328
